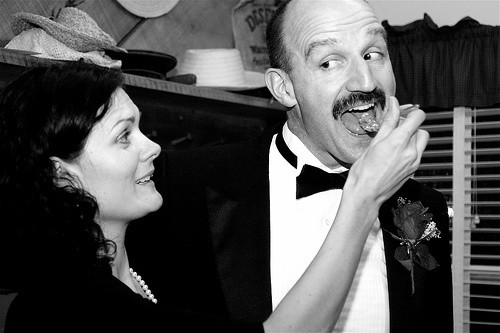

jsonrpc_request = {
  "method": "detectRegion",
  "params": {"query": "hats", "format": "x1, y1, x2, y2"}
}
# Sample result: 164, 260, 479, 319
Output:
11, 6, 128, 54
103, 49, 177, 79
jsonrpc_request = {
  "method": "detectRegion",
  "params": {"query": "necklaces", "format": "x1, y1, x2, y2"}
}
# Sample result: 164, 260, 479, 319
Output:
129, 268, 157, 304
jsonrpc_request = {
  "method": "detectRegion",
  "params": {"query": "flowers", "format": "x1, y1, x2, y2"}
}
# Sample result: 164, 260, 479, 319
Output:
386, 197, 441, 294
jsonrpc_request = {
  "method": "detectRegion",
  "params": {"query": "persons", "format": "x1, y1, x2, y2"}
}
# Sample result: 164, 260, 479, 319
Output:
0, 61, 430, 333
152, 0, 453, 333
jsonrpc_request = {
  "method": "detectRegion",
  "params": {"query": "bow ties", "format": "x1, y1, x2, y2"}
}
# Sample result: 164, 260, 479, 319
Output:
275, 127, 349, 199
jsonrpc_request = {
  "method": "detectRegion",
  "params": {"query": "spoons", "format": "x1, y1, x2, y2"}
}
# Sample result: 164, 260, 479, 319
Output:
359, 104, 420, 131
